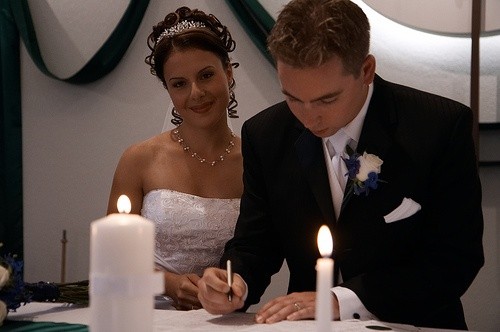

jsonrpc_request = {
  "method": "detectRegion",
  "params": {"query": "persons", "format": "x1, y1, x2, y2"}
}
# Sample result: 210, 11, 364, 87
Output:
106, 6, 244, 310
196, 0, 485, 330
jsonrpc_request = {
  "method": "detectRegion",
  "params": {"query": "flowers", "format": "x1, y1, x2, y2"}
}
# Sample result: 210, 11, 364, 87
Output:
0, 244, 88, 325
340, 151, 387, 209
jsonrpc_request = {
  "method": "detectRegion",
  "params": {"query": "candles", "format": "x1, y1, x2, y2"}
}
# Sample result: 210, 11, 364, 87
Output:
316, 224, 334, 332
89, 194, 155, 332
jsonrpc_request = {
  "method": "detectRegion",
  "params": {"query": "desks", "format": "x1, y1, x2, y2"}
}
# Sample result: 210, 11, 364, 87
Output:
7, 303, 465, 332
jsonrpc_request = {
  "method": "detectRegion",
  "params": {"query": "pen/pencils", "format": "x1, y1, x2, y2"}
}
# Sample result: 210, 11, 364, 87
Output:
227, 261, 232, 302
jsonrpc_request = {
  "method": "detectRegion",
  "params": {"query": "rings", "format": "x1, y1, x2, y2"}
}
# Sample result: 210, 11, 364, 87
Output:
295, 303, 301, 311
192, 306, 196, 310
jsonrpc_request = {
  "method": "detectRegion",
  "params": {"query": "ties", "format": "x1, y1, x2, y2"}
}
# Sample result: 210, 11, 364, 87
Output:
329, 131, 352, 194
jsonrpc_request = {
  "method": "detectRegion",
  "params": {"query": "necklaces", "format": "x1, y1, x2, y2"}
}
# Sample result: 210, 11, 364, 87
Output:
173, 127, 236, 167
